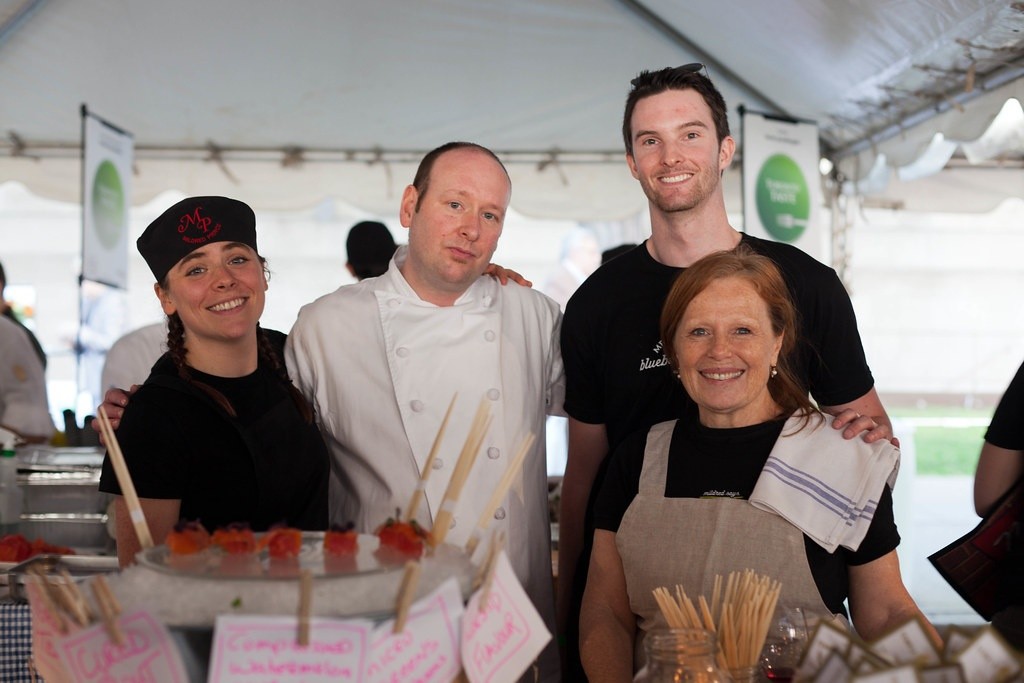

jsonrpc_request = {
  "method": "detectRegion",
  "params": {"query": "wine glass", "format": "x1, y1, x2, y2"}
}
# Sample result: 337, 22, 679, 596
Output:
759, 605, 810, 683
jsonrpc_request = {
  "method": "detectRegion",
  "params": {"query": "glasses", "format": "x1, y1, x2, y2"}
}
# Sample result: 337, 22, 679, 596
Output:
630, 63, 709, 92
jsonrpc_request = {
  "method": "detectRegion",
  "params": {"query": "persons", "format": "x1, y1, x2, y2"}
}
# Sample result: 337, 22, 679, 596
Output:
91, 67, 946, 682
973, 363, 1024, 517
1, 263, 57, 442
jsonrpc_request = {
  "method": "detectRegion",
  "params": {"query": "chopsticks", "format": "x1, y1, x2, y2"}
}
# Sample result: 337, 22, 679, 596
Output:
651, 569, 782, 673
296, 392, 536, 648
22, 561, 127, 648
93, 405, 155, 550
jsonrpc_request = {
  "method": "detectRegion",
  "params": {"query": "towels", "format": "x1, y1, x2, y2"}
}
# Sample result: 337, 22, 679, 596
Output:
748, 405, 901, 556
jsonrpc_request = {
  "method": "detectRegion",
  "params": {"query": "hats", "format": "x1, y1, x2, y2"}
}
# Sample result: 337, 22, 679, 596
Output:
137, 196, 257, 283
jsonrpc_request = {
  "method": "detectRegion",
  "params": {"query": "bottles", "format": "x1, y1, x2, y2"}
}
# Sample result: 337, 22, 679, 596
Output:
727, 661, 772, 683
633, 629, 733, 683
